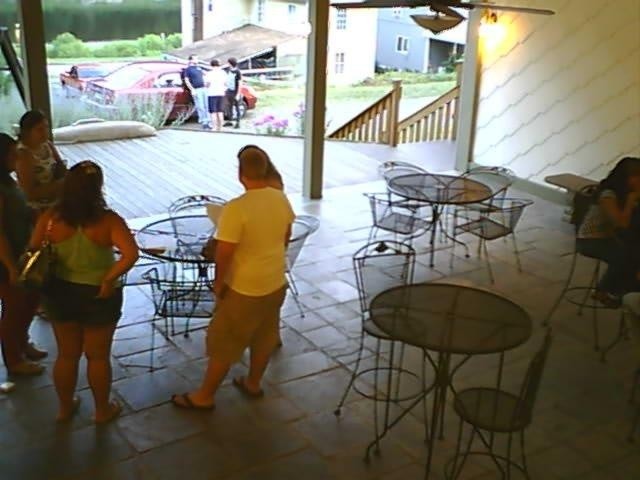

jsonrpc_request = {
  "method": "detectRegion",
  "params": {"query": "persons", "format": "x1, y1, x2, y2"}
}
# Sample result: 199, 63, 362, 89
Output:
237, 145, 283, 191
0, 133, 48, 374
171, 148, 296, 410
185, 51, 212, 128
577, 156, 640, 309
26, 160, 139, 425
222, 57, 241, 130
202, 59, 229, 131
15, 110, 70, 319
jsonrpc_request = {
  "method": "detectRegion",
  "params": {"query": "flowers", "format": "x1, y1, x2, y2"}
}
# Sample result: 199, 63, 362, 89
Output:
293, 102, 332, 137
253, 115, 291, 136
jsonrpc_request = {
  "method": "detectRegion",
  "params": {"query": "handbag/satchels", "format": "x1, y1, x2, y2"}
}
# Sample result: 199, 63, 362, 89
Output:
33, 211, 59, 321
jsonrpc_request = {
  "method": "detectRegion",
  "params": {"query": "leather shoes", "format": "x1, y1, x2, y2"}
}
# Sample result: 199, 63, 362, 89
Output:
7, 360, 44, 375
28, 344, 49, 360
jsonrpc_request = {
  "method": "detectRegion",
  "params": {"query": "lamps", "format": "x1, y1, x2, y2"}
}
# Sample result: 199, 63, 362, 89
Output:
411, 10, 466, 34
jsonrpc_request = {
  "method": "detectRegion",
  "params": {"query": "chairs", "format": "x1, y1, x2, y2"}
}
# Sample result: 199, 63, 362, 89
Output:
110, 228, 181, 336
362, 191, 434, 279
444, 325, 553, 480
441, 166, 518, 259
139, 268, 222, 372
280, 215, 320, 318
334, 237, 451, 458
378, 160, 450, 247
168, 194, 230, 307
622, 292, 640, 444
448, 197, 534, 285
542, 184, 628, 366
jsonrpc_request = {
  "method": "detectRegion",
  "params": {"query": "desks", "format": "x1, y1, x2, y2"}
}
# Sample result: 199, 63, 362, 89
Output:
362, 282, 533, 480
136, 213, 216, 338
387, 173, 492, 267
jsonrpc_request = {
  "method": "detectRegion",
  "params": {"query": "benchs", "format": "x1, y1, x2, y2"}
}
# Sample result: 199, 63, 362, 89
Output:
543, 172, 599, 222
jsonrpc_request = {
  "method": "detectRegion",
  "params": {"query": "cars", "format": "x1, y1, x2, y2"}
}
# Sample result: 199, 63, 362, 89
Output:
80, 62, 257, 119
60, 65, 104, 91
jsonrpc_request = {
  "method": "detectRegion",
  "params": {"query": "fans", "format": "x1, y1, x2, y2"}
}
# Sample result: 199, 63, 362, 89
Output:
329, 1, 554, 17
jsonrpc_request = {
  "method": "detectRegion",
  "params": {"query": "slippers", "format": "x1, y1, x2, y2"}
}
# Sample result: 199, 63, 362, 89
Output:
232, 374, 265, 399
55, 395, 82, 422
171, 393, 217, 412
95, 396, 122, 423
